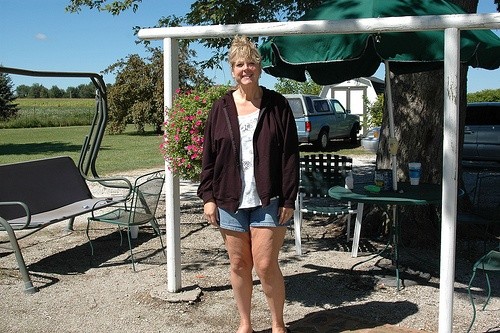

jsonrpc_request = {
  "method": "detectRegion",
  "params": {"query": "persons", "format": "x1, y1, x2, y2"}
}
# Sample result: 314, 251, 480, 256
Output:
197, 36, 300, 333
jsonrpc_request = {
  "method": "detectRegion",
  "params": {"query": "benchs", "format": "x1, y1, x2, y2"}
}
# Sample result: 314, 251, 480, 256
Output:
0, 67, 159, 293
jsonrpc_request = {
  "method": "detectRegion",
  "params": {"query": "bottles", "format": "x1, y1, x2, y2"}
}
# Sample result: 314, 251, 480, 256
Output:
345, 173, 353, 189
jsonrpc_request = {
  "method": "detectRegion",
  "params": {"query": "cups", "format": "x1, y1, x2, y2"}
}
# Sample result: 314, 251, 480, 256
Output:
408, 163, 421, 186
130, 226, 139, 239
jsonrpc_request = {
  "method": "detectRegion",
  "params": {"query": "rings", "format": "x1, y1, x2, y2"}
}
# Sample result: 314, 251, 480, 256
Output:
208, 222, 210, 224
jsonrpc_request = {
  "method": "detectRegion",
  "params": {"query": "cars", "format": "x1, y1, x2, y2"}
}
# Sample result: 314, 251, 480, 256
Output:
361, 101, 500, 163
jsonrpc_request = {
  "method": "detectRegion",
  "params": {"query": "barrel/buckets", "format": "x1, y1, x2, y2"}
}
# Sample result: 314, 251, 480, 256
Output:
375, 169, 393, 191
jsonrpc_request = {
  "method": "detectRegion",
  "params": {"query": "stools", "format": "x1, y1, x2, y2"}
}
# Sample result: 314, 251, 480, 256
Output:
467, 249, 500, 333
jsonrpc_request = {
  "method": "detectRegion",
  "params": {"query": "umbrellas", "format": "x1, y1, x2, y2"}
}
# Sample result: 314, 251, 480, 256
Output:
257, 0, 500, 262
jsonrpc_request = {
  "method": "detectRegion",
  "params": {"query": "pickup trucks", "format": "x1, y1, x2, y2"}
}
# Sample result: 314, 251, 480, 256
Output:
282, 93, 361, 151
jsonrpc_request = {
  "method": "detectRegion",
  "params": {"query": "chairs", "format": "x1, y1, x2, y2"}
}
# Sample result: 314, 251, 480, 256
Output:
86, 170, 165, 272
293, 156, 354, 246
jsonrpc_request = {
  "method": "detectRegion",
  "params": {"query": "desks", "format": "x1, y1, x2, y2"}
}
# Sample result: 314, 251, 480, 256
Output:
328, 182, 465, 290
